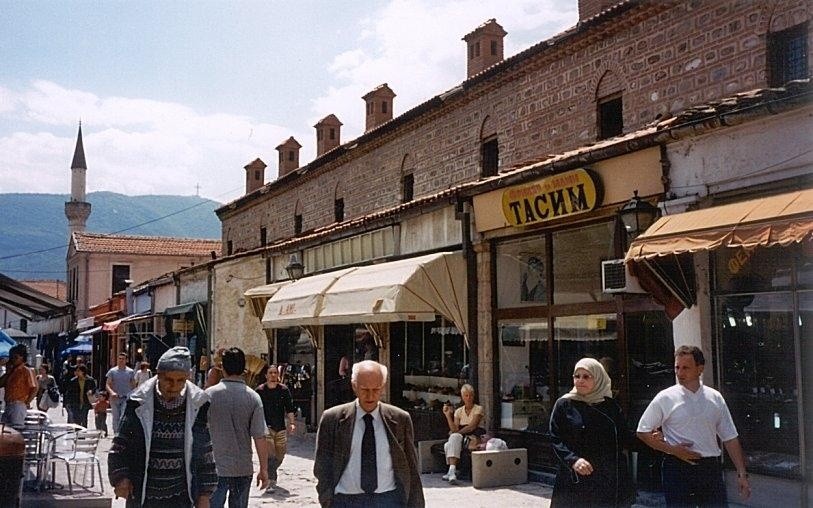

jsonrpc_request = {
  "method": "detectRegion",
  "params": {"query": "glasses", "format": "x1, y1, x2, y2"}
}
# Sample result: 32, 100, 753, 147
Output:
573, 374, 594, 380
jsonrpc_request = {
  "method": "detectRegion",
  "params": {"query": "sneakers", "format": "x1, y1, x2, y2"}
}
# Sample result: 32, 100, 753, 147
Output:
443, 471, 462, 480
449, 473, 456, 485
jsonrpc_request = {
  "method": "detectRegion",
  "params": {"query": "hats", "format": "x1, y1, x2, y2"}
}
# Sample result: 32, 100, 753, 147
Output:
157, 346, 191, 373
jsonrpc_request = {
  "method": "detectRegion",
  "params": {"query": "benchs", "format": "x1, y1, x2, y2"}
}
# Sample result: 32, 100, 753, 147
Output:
419, 439, 531, 489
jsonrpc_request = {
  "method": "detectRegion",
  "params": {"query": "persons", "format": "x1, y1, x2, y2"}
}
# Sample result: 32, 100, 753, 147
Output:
204, 348, 268, 508
443, 383, 484, 482
254, 366, 296, 491
60, 355, 72, 397
314, 361, 426, 507
0, 344, 35, 431
550, 358, 640, 508
73, 357, 84, 372
134, 361, 153, 388
65, 365, 97, 431
109, 347, 219, 508
202, 348, 227, 390
94, 392, 110, 439
0, 357, 6, 415
339, 353, 353, 405
38, 378, 61, 411
105, 353, 134, 438
636, 346, 752, 507
35, 364, 56, 413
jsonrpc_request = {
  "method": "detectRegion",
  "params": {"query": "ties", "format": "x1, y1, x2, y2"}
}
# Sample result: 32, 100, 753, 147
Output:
361, 415, 378, 493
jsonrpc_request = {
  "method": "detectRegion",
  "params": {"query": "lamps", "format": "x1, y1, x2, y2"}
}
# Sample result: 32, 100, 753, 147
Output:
614, 189, 661, 240
286, 251, 305, 284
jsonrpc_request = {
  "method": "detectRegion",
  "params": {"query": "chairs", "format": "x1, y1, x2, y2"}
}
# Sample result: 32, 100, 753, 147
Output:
47, 423, 79, 492
9, 428, 46, 492
55, 428, 105, 495
23, 408, 50, 480
